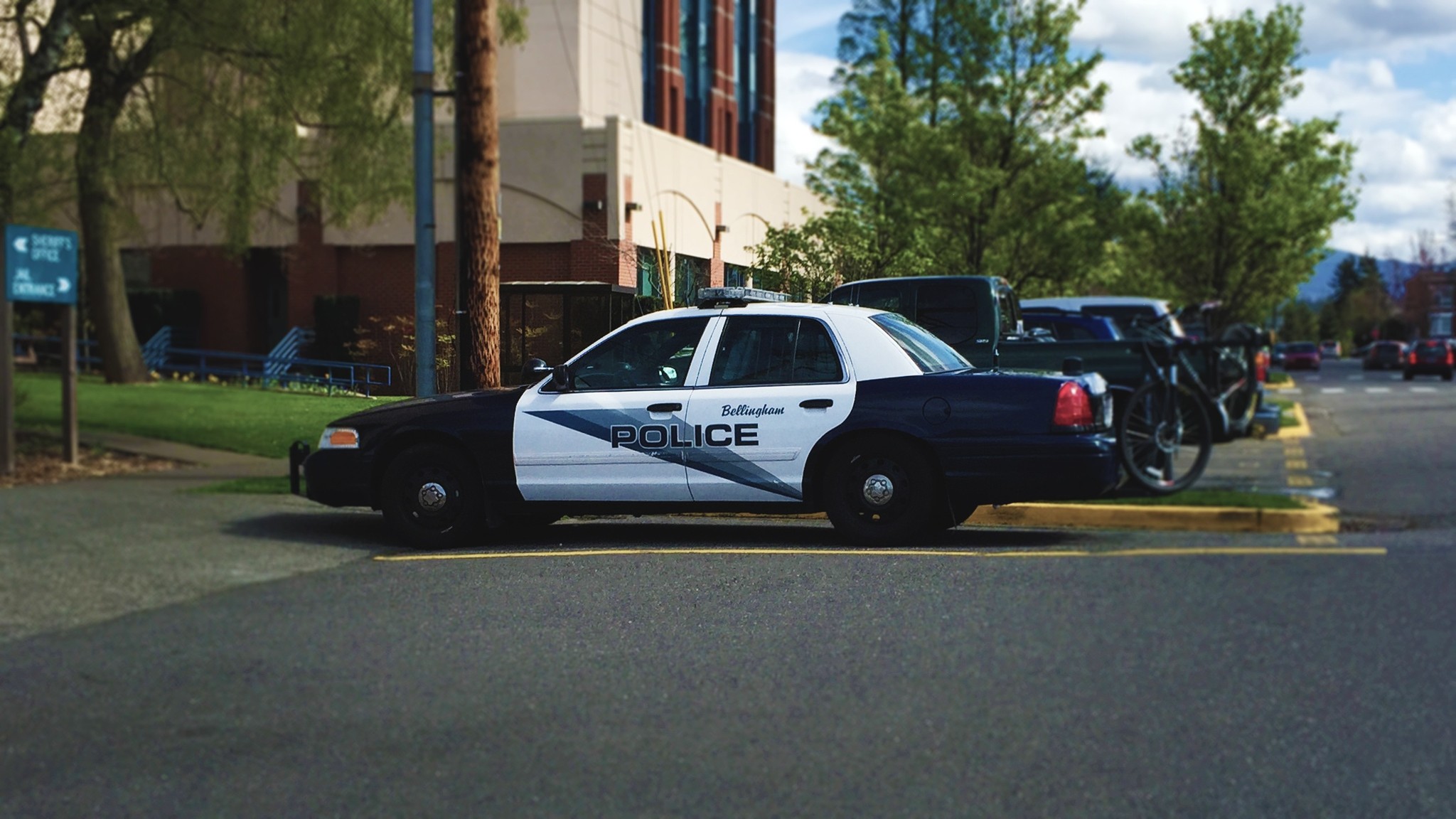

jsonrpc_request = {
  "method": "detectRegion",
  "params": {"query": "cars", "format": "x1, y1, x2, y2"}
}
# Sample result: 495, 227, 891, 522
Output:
1277, 341, 1322, 367
1319, 340, 1342, 358
1363, 337, 1456, 381
288, 286, 1122, 550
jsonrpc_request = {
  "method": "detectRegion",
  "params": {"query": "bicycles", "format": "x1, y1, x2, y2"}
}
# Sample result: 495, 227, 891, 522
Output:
1120, 299, 1270, 496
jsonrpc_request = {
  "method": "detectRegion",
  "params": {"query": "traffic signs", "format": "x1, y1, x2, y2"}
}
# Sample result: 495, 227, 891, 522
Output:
4, 225, 79, 303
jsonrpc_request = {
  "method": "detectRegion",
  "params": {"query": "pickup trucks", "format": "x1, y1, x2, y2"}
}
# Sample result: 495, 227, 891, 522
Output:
817, 274, 1261, 468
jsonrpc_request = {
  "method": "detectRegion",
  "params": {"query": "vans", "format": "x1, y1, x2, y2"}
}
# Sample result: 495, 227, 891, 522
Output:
1017, 295, 1187, 358
